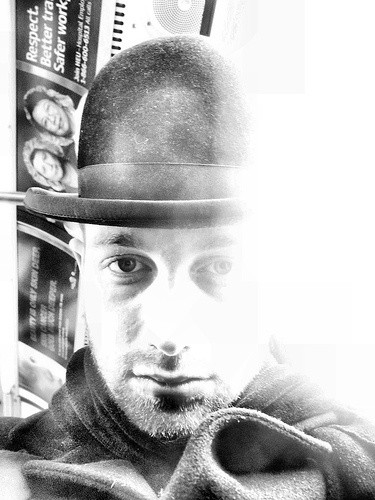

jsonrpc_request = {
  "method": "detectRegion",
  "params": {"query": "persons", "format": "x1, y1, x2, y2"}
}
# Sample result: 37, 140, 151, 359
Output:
0, 35, 375, 499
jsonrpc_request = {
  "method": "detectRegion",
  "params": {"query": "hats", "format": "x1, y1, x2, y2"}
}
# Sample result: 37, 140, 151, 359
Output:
25, 36, 266, 228
